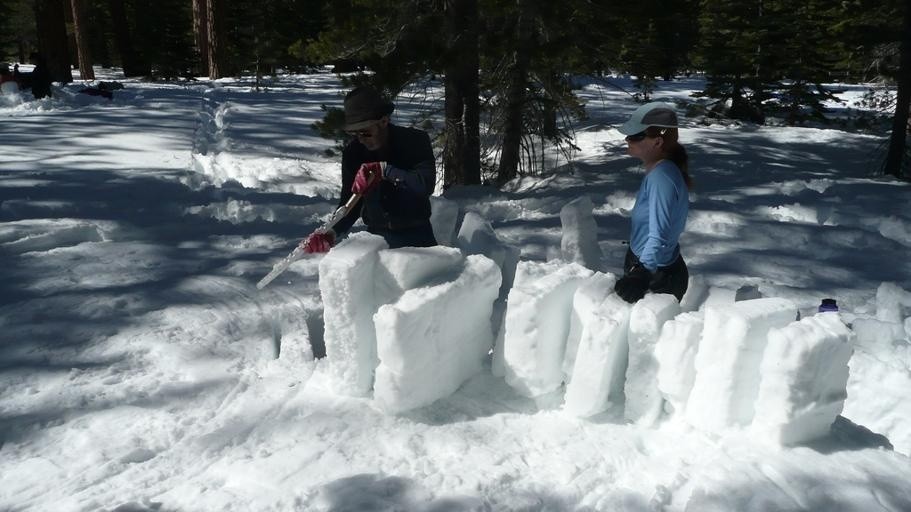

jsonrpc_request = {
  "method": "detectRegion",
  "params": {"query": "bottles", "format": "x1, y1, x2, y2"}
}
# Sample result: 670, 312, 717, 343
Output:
819, 298, 839, 313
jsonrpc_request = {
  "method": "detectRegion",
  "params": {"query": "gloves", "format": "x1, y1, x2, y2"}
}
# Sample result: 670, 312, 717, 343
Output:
615, 265, 648, 303
303, 234, 331, 253
351, 162, 382, 195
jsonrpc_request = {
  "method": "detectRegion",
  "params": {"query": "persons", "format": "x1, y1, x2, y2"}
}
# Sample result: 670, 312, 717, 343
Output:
611, 100, 690, 305
303, 87, 437, 254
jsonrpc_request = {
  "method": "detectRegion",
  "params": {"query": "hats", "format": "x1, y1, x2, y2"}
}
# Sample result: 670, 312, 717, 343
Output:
618, 102, 680, 135
342, 87, 394, 131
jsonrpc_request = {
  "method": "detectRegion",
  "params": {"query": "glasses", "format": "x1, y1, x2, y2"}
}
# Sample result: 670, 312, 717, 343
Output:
630, 132, 646, 143
349, 131, 371, 138
